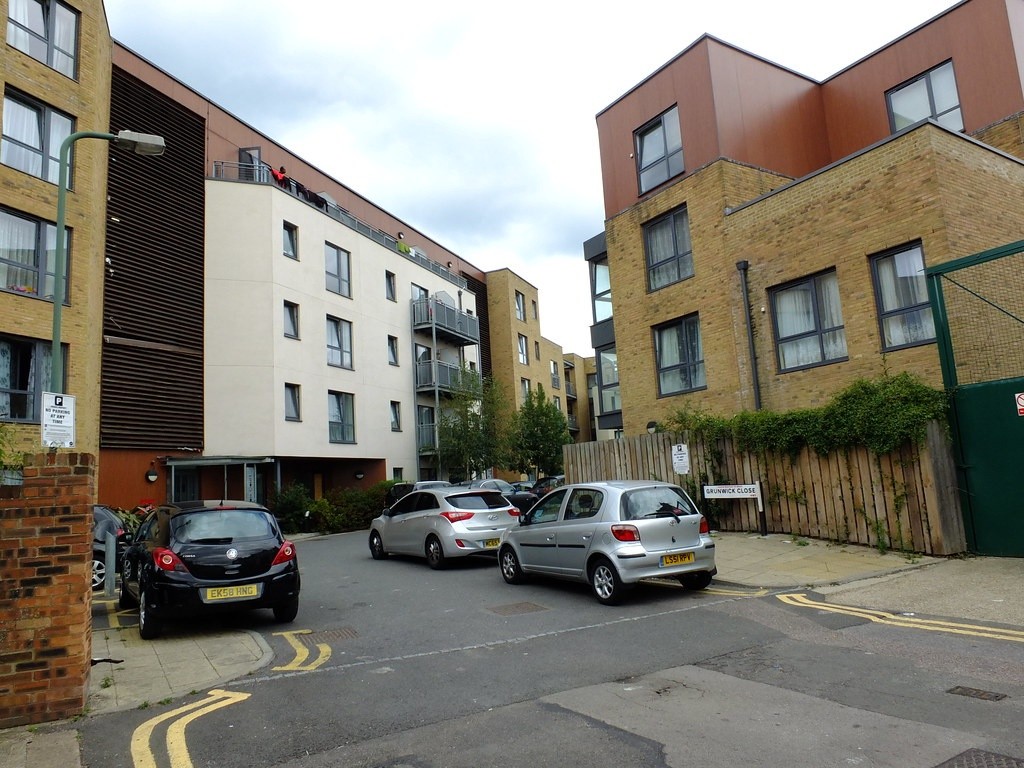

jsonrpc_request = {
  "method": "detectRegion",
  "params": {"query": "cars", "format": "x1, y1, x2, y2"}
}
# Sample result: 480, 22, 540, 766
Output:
497, 480, 717, 606
448, 479, 539, 516
92, 504, 132, 591
529, 477, 564, 500
368, 486, 521, 570
510, 481, 536, 492
119, 500, 301, 641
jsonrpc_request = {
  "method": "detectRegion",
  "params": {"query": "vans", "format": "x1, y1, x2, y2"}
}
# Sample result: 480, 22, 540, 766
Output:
383, 481, 452, 512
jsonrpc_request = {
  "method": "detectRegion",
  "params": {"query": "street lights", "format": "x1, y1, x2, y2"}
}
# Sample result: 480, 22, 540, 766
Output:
48, 129, 166, 454
